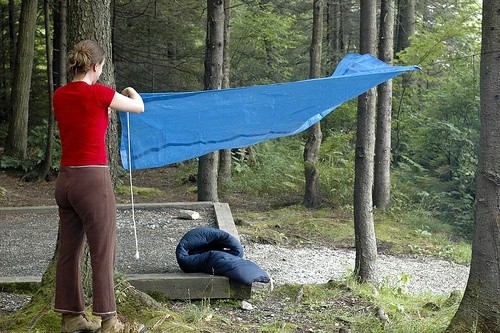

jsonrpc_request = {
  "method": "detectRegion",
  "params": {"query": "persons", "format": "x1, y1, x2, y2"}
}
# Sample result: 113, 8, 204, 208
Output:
53, 39, 147, 333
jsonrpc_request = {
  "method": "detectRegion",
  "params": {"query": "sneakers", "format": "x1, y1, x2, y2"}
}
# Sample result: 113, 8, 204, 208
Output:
60, 314, 148, 333
